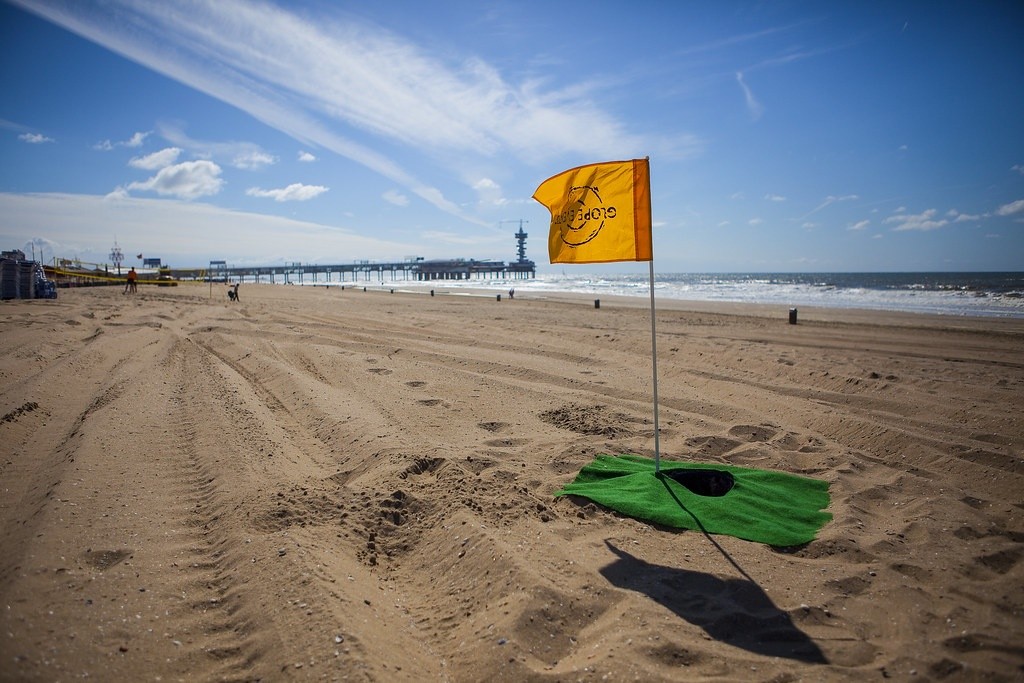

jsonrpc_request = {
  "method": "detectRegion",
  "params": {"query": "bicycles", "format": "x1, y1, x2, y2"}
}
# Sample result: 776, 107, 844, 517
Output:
508, 293, 514, 299
127, 278, 138, 294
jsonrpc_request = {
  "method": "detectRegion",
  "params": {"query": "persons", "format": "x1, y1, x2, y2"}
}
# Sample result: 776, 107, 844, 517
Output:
233, 283, 240, 301
122, 266, 138, 294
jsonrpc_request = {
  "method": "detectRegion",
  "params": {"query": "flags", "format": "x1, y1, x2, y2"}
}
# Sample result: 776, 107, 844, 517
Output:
531, 158, 653, 264
